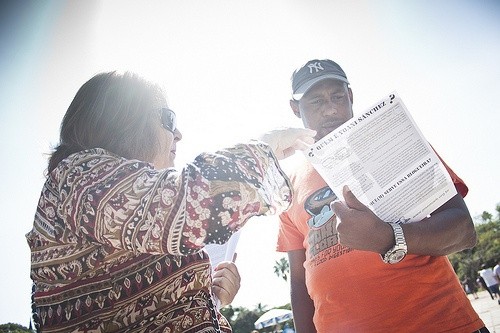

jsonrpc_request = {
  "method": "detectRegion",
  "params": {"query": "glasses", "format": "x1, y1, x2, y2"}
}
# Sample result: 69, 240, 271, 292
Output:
160, 106, 176, 131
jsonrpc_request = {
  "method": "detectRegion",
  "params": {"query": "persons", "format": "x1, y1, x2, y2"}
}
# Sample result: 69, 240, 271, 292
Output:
462, 259, 500, 306
25, 70, 318, 333
260, 59, 490, 333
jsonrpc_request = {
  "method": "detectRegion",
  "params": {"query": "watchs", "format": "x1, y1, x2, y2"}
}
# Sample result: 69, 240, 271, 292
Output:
379, 221, 407, 264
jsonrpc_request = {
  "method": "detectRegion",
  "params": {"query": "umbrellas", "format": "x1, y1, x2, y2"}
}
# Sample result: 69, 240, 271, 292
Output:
254, 308, 293, 333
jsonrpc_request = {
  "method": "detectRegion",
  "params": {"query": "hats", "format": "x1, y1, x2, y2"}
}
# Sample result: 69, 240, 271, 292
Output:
292, 59, 350, 101
